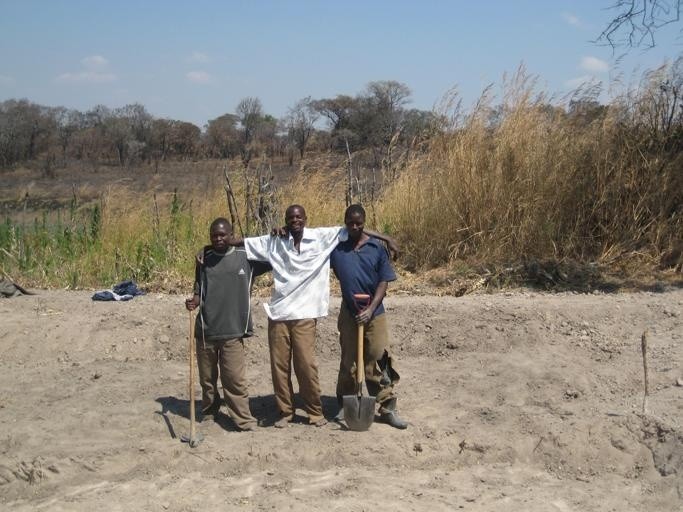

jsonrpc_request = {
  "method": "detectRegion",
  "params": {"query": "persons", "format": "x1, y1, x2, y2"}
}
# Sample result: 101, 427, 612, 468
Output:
329, 203, 408, 428
196, 204, 399, 428
183, 217, 289, 430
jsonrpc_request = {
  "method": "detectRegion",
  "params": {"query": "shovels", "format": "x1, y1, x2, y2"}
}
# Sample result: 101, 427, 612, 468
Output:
342, 293, 376, 431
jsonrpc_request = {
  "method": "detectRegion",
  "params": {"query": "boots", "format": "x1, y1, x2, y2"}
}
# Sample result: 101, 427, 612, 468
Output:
380, 413, 407, 429
